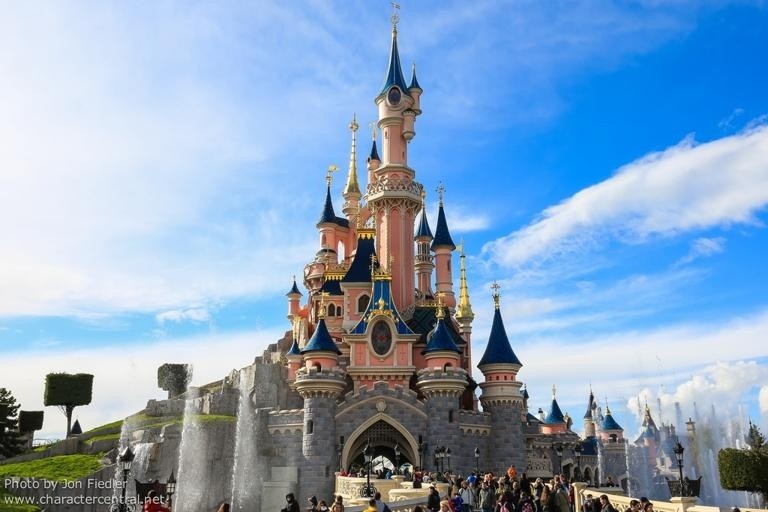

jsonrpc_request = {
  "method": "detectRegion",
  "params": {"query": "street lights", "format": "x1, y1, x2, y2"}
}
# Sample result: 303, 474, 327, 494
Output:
393, 441, 402, 475
359, 437, 378, 497
575, 442, 581, 477
109, 438, 135, 512
418, 448, 423, 470
336, 447, 341, 472
672, 437, 685, 496
434, 445, 451, 472
474, 447, 480, 474
556, 443, 564, 475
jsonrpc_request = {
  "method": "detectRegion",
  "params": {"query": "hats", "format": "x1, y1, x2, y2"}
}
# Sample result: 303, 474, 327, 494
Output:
508, 469, 516, 479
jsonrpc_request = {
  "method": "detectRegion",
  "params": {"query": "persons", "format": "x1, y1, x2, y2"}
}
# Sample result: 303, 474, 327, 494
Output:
305, 461, 653, 512
143, 490, 172, 512
280, 493, 300, 512
217, 503, 229, 512
